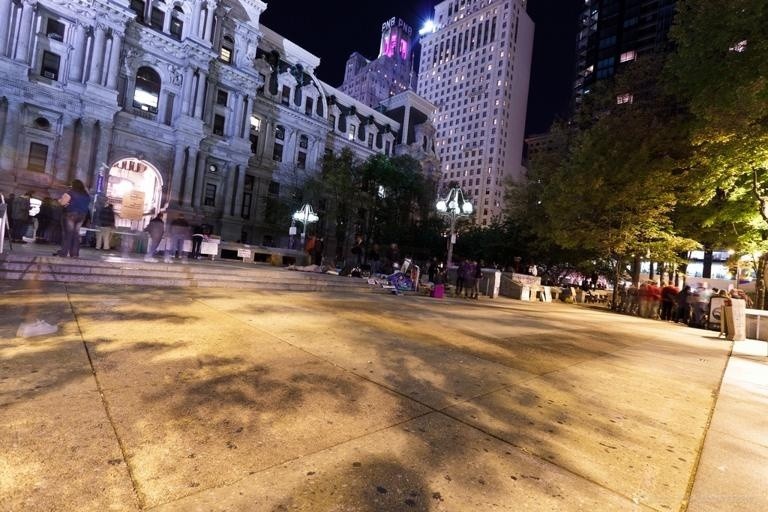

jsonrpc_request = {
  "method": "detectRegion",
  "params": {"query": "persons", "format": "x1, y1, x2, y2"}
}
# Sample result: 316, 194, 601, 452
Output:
191, 224, 206, 261
426, 255, 439, 282
144, 212, 166, 263
168, 213, 191, 258
315, 235, 325, 266
616, 280, 752, 328
7, 180, 96, 259
370, 244, 381, 275
456, 258, 483, 298
391, 242, 401, 270
93, 204, 120, 252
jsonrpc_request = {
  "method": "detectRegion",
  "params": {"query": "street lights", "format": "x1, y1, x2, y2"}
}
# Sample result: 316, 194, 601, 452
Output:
436, 188, 474, 266
292, 203, 319, 254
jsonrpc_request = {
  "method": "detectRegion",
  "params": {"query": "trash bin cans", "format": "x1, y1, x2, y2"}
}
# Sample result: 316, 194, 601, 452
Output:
722, 297, 748, 342
